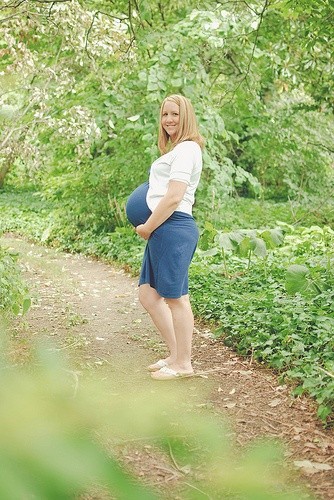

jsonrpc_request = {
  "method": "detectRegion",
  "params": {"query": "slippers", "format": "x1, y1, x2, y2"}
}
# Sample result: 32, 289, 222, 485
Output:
151, 366, 195, 381
148, 359, 168, 372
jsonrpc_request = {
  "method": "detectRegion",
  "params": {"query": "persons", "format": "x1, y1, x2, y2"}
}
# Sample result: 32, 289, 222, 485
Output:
125, 95, 203, 381
